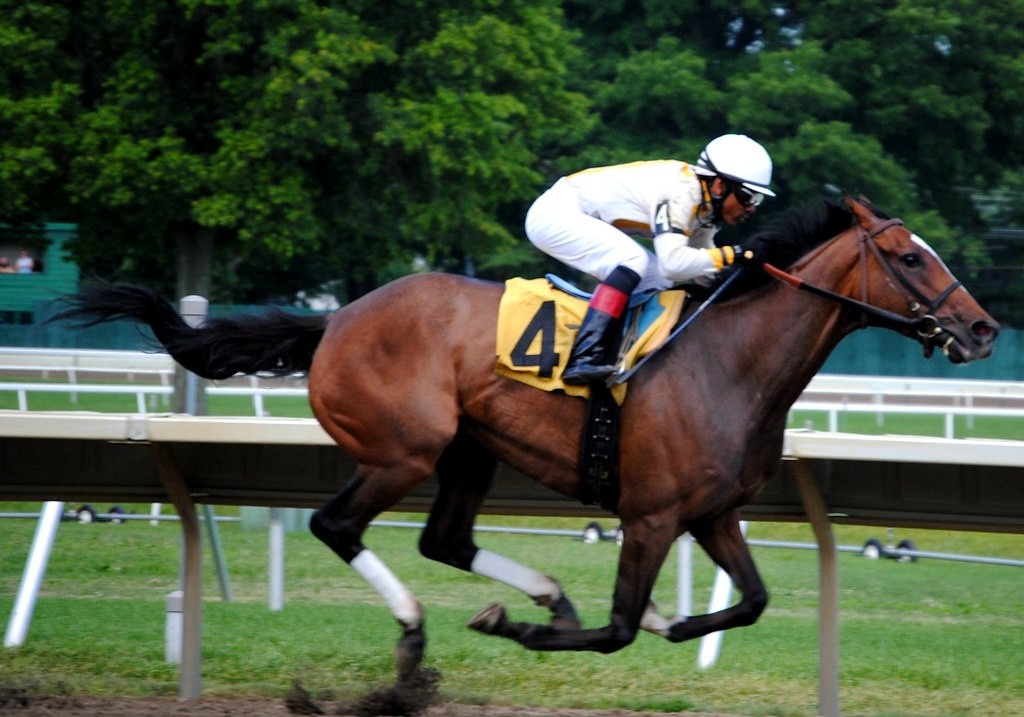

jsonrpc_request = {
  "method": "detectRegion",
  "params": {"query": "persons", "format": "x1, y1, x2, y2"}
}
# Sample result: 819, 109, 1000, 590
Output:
525, 134, 778, 386
0, 257, 14, 273
14, 249, 33, 274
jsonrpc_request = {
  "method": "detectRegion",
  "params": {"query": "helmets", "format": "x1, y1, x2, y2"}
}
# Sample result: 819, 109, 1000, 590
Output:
693, 133, 778, 198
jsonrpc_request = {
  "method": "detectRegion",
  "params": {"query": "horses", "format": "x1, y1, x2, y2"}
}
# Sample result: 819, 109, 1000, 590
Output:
39, 186, 1000, 680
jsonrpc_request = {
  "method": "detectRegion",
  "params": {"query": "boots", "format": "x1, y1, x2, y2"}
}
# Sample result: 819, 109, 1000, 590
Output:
561, 265, 642, 385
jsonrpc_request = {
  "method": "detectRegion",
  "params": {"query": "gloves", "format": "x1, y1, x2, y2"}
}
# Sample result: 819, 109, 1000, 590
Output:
733, 231, 786, 269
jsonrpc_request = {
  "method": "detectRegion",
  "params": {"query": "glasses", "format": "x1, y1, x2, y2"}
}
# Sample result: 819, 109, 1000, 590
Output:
717, 172, 764, 208
